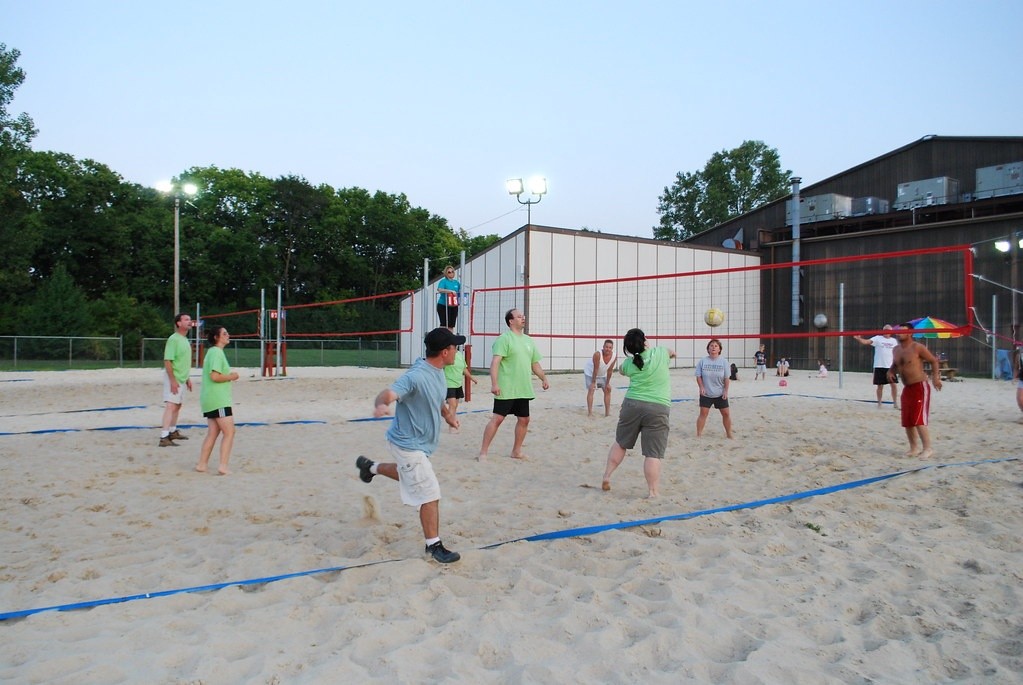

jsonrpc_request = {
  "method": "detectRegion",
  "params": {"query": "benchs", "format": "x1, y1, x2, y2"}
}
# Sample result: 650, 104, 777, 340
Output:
923, 368, 958, 380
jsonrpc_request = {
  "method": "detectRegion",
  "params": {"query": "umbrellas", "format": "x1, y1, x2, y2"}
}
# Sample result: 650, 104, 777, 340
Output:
892, 316, 966, 350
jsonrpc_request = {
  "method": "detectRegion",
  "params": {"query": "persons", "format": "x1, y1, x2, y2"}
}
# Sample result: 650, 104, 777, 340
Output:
887, 322, 942, 458
854, 325, 899, 408
694, 339, 734, 440
754, 344, 767, 380
477, 308, 550, 461
602, 329, 677, 498
356, 327, 466, 563
436, 264, 460, 333
583, 340, 618, 416
158, 313, 193, 446
195, 325, 239, 474
1013, 348, 1023, 424
808, 360, 828, 378
444, 345, 477, 434
777, 357, 789, 377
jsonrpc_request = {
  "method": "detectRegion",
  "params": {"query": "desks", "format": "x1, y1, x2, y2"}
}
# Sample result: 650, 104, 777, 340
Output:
923, 359, 949, 369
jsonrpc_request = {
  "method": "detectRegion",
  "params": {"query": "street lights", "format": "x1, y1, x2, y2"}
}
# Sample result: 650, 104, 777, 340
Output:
157, 180, 197, 333
506, 175, 546, 226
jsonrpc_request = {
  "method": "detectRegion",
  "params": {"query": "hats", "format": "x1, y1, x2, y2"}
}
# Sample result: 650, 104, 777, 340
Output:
424, 328, 466, 356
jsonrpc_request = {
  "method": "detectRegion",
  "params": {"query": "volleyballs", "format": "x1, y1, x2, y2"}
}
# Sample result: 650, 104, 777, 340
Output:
703, 307, 724, 327
814, 313, 827, 328
779, 380, 787, 386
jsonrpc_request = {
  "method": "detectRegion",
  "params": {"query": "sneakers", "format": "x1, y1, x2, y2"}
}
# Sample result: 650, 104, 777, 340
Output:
424, 541, 459, 563
356, 455, 375, 483
159, 435, 180, 447
170, 429, 187, 440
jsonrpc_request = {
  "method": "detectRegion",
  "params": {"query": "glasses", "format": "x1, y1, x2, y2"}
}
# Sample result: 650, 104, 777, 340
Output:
448, 271, 454, 274
